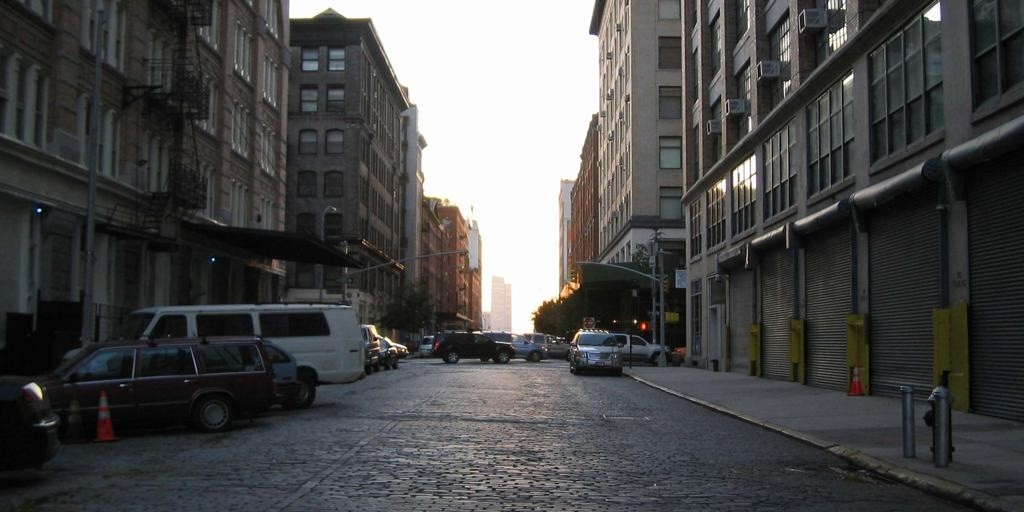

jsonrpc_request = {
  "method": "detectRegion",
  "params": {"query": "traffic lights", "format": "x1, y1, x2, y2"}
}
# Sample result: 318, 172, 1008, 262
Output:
640, 323, 646, 330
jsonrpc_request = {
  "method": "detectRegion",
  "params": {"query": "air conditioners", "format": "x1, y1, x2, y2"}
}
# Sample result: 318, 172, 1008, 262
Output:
795, 8, 828, 35
704, 96, 745, 136
755, 59, 780, 82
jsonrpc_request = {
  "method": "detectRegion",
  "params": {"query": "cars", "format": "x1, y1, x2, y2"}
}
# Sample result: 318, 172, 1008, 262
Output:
420, 335, 434, 356
1, 373, 57, 471
612, 333, 672, 365
358, 324, 410, 375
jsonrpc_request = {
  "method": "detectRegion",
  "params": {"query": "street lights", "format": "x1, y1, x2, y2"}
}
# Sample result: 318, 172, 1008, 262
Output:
318, 205, 337, 301
613, 320, 616, 330
629, 319, 637, 368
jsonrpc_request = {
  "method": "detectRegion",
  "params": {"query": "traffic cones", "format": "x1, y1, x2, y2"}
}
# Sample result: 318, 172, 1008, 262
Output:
848, 361, 866, 396
96, 390, 119, 441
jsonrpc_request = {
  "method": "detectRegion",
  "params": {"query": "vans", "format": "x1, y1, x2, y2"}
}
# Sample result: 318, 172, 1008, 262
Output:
35, 335, 279, 432
105, 299, 366, 409
484, 333, 543, 362
526, 334, 571, 361
569, 331, 623, 375
432, 331, 515, 363
